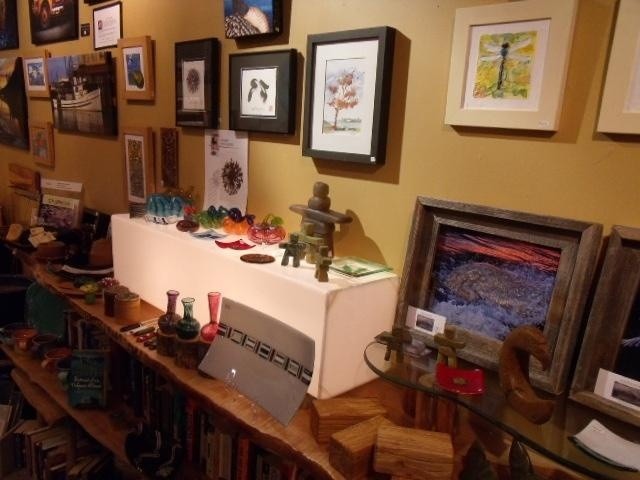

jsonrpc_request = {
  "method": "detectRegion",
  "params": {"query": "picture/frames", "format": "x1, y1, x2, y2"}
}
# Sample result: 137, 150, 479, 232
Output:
394, 195, 602, 395
567, 224, 639, 431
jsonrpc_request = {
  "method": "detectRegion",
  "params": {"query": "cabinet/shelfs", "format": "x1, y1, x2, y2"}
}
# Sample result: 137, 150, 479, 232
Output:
0, 258, 639, 479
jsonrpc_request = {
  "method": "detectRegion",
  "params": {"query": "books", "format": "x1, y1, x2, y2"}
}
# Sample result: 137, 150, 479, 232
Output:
63, 312, 316, 480
13, 420, 62, 480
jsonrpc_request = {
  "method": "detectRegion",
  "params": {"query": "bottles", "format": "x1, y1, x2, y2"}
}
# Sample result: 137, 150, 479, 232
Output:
158, 290, 182, 335
175, 297, 201, 340
200, 292, 222, 342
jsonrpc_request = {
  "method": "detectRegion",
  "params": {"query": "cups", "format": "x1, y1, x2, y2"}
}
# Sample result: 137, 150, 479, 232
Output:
11, 329, 73, 390
